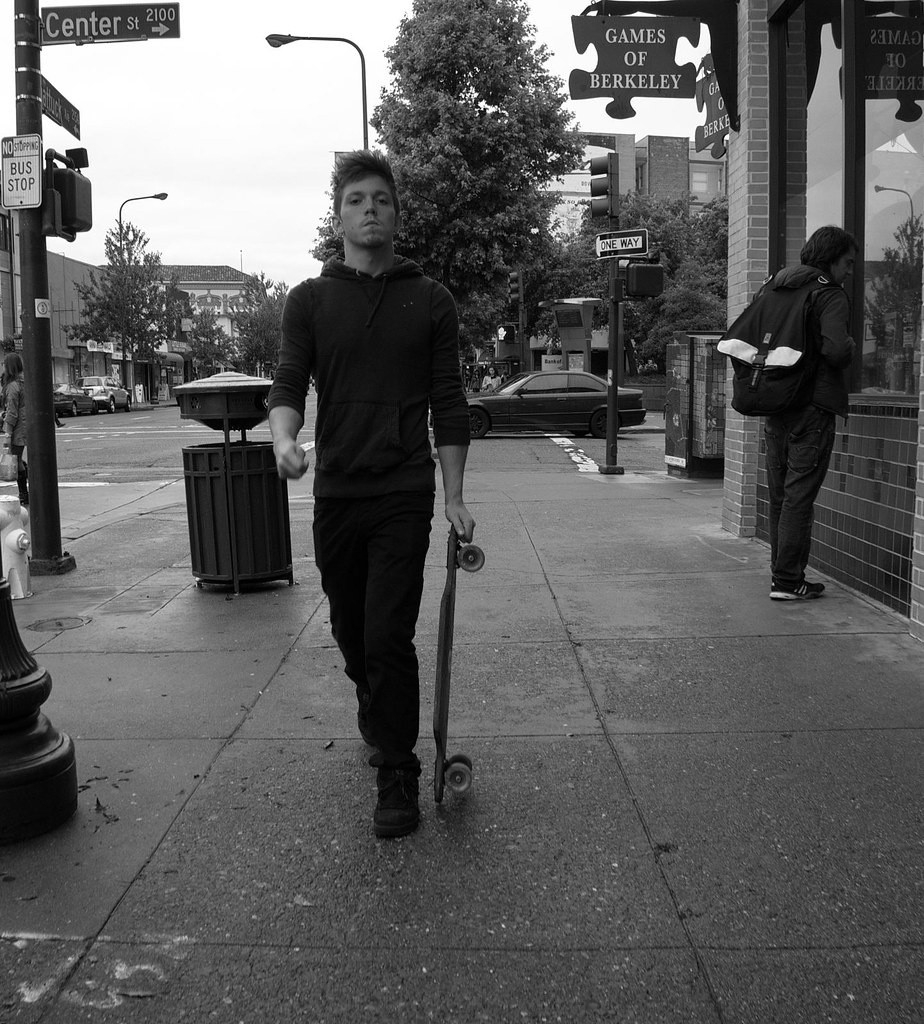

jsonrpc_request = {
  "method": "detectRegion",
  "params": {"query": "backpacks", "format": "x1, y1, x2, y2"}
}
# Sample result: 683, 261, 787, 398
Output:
715, 269, 843, 418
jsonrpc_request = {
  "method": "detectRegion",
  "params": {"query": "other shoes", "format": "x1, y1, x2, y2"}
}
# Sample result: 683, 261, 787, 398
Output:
368, 750, 423, 839
57, 423, 66, 428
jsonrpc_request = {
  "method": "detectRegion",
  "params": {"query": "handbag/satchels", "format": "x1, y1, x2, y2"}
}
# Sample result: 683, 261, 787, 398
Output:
0, 446, 18, 481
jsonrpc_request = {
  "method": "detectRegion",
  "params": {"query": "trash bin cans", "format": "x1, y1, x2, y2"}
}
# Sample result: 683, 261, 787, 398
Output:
172, 371, 293, 596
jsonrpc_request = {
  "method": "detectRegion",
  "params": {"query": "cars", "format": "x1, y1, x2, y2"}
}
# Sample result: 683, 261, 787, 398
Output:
75, 375, 133, 413
52, 382, 99, 416
428, 368, 648, 438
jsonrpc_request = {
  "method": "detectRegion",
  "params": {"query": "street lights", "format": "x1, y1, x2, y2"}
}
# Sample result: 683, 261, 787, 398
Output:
266, 33, 369, 150
874, 185, 914, 218
120, 193, 169, 389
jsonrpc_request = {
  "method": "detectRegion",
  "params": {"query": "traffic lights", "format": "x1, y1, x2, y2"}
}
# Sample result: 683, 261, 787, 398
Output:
498, 325, 515, 341
508, 270, 523, 304
590, 153, 620, 217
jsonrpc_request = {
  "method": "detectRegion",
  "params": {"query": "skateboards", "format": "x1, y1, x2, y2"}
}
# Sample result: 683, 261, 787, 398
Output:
429, 524, 486, 808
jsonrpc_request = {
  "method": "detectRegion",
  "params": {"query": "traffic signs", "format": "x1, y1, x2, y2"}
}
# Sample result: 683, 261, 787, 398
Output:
596, 228, 649, 260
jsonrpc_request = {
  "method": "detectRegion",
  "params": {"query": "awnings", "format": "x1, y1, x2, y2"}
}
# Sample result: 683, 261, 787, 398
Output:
132, 351, 184, 367
215, 358, 237, 369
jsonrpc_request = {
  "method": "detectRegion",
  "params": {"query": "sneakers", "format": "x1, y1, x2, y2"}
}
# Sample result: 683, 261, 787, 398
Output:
770, 574, 826, 600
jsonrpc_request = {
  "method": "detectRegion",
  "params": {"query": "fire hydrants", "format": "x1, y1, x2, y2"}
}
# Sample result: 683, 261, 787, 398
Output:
0, 494, 34, 599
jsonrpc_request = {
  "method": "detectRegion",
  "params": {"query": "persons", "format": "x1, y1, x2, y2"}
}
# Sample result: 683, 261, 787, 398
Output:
263, 151, 478, 838
1, 353, 30, 507
473, 366, 508, 393
747, 224, 860, 602
55, 413, 66, 428
